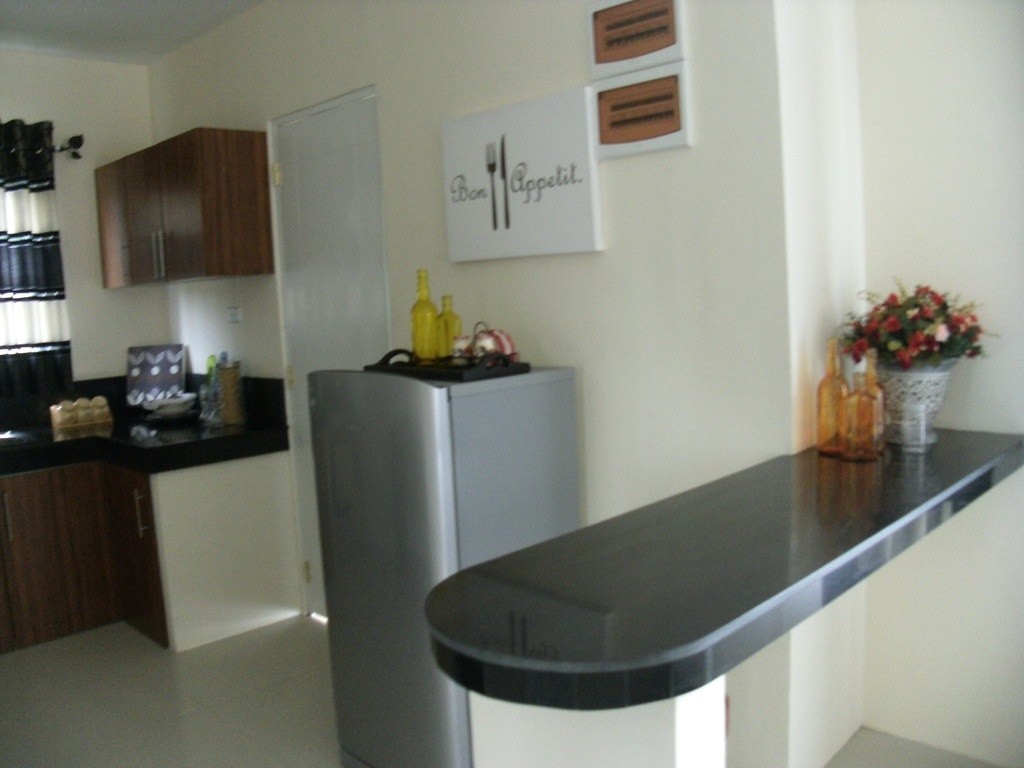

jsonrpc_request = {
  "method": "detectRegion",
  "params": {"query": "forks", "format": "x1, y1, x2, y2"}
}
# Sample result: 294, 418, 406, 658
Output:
486, 141, 498, 231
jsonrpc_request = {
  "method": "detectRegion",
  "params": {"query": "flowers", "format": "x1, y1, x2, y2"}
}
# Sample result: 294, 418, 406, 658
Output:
836, 278, 992, 372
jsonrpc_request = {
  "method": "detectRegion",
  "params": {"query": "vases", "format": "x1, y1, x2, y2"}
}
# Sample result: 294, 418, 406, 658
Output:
876, 359, 959, 446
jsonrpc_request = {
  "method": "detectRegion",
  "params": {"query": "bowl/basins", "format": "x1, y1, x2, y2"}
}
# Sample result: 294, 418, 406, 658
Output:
142, 391, 197, 413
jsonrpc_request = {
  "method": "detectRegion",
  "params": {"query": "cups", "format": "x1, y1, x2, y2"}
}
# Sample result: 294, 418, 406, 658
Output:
217, 368, 241, 425
902, 402, 926, 453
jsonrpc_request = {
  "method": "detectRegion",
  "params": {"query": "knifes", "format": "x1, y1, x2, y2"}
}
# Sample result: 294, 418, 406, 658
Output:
500, 133, 511, 229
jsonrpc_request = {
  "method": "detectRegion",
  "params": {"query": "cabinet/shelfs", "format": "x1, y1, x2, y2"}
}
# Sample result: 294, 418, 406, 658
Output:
0, 410, 307, 656
95, 126, 274, 290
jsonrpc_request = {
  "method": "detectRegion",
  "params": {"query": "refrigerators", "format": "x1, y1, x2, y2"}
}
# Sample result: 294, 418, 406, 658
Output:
309, 371, 581, 768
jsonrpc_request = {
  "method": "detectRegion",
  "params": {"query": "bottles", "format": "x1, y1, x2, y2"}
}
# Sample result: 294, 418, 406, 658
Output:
438, 295, 462, 357
58, 396, 112, 437
411, 268, 438, 359
816, 337, 885, 459
